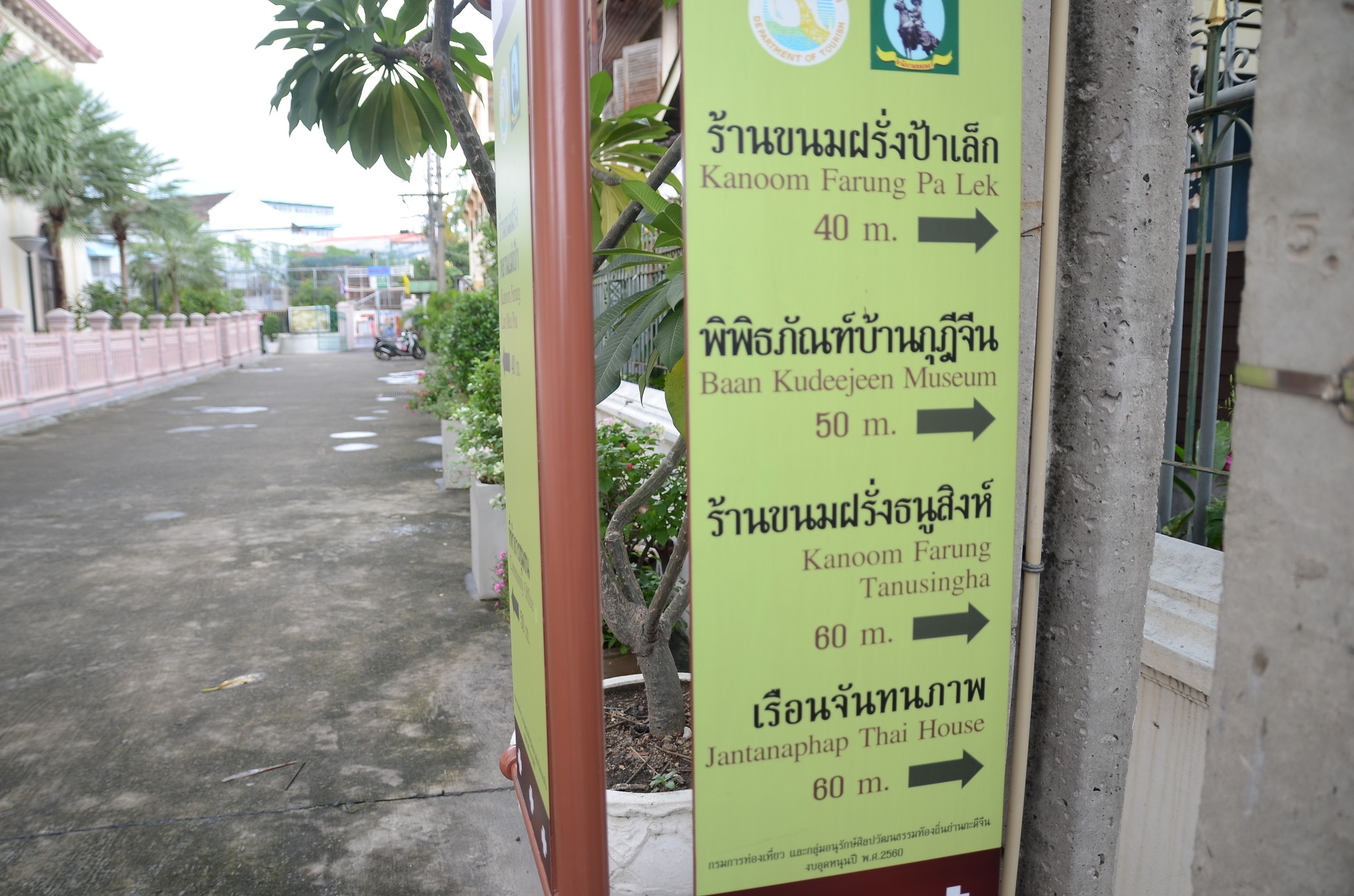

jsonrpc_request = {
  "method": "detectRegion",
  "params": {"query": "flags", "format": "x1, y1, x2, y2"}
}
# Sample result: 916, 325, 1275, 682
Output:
403, 273, 411, 295
338, 274, 350, 295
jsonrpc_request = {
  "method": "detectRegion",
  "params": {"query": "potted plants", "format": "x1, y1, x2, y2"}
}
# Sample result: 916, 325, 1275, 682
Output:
253, 0, 721, 894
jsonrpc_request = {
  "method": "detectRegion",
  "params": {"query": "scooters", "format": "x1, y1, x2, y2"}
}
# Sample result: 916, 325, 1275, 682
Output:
374, 327, 426, 360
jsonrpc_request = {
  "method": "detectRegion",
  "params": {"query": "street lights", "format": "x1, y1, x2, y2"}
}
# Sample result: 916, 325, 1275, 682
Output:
143, 262, 169, 313
9, 236, 48, 331
